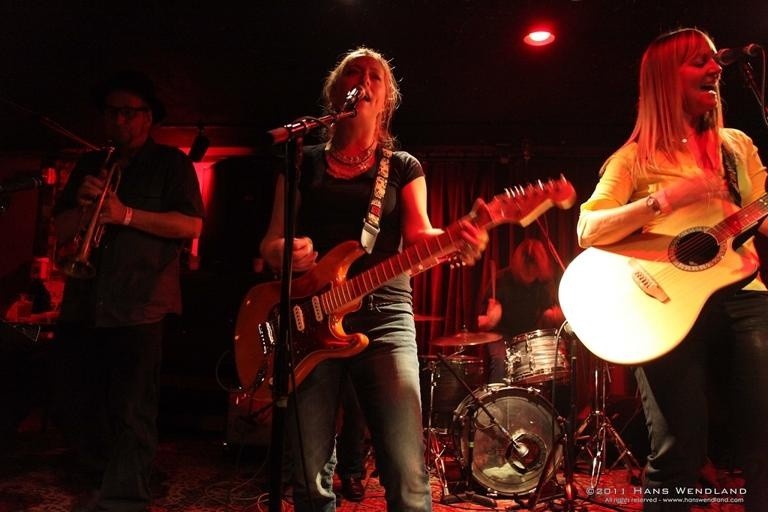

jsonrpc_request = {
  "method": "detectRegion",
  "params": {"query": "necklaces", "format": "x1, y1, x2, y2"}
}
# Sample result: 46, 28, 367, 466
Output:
671, 128, 698, 149
326, 134, 377, 167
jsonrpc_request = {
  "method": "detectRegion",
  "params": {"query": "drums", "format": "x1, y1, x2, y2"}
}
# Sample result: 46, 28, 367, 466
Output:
416, 354, 487, 414
453, 387, 571, 501
504, 327, 573, 385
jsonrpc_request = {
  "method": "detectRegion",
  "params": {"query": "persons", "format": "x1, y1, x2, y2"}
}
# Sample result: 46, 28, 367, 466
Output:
577, 28, 768, 512
50, 81, 207, 510
259, 47, 488, 512
477, 240, 567, 405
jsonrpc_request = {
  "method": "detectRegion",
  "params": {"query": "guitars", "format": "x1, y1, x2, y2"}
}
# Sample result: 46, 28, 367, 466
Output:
233, 174, 579, 404
556, 190, 768, 368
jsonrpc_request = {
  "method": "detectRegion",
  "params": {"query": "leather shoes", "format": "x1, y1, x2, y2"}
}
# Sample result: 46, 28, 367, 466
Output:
342, 473, 364, 501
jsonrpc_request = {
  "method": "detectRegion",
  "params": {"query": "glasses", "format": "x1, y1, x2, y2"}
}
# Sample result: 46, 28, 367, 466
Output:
104, 105, 149, 119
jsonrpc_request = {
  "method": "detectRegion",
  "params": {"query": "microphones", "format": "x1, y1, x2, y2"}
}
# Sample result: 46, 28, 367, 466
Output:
347, 85, 365, 107
0, 172, 56, 193
716, 45, 760, 64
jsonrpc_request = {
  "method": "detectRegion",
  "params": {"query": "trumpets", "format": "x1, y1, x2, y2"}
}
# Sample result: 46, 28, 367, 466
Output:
59, 145, 120, 283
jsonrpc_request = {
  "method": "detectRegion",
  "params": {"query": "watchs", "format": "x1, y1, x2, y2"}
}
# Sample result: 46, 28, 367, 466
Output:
122, 206, 136, 226
647, 193, 672, 217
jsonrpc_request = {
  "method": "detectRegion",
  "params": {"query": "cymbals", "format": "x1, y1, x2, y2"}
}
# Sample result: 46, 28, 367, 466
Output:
431, 324, 503, 346
